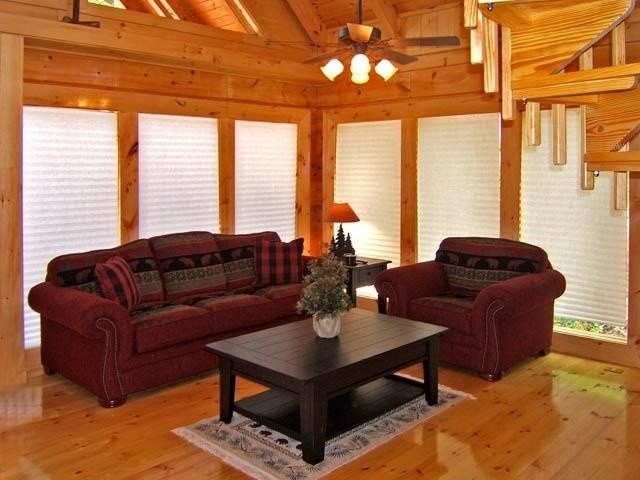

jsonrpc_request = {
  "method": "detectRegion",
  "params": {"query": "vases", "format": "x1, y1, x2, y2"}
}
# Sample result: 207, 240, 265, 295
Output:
313, 309, 340, 340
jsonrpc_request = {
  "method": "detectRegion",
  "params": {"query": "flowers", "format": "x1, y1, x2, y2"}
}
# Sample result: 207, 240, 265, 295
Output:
295, 247, 351, 315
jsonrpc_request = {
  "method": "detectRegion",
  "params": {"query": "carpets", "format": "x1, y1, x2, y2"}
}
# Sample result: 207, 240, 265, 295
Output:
169, 371, 473, 480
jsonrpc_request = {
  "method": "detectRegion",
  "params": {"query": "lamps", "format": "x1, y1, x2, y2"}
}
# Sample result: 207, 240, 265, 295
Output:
319, 52, 400, 85
320, 202, 357, 261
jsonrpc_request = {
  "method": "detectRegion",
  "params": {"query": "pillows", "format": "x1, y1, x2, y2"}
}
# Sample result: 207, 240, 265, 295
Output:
96, 256, 141, 310
255, 237, 305, 285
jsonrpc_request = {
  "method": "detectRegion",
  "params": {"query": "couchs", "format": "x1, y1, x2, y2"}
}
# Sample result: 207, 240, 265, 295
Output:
29, 231, 310, 408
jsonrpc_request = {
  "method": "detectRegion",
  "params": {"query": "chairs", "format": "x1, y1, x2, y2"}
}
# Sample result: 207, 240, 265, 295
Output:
374, 237, 564, 382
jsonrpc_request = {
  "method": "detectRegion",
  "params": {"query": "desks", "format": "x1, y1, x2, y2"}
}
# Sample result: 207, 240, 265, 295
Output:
322, 250, 390, 312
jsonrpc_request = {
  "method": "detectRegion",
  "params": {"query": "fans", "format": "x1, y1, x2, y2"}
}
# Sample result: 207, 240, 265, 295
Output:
302, 0, 460, 80
